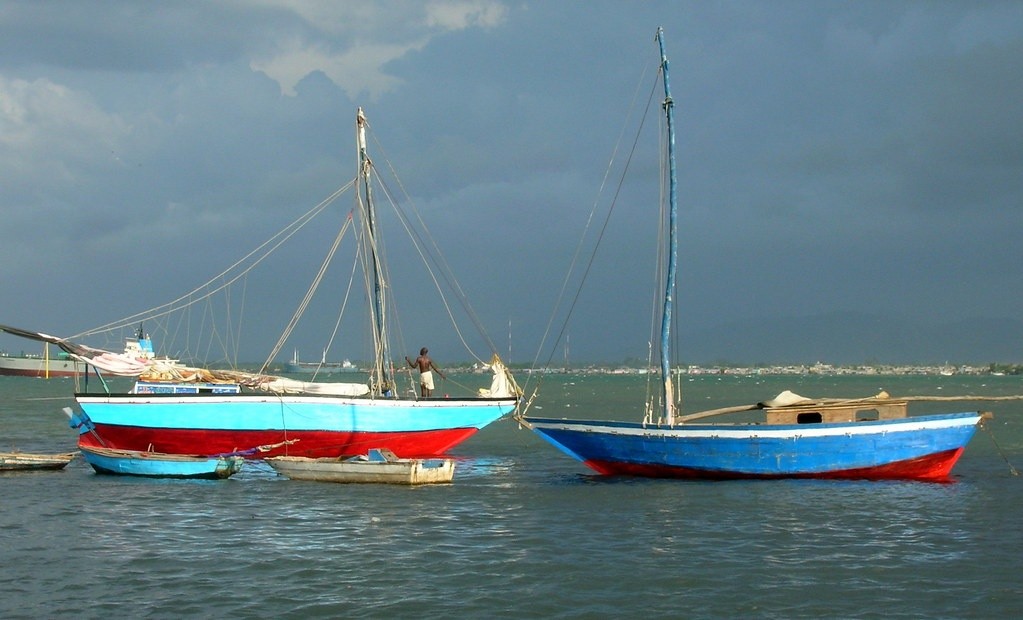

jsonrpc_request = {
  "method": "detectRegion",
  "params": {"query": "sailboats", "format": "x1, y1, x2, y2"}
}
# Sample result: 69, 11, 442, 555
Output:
73, 109, 521, 461
510, 24, 984, 483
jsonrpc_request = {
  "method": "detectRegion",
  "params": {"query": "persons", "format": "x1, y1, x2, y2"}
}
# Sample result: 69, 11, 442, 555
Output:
405, 347, 446, 398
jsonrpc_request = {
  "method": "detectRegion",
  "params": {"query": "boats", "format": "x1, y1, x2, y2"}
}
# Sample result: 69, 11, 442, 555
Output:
264, 446, 457, 486
0, 355, 113, 374
0, 453, 75, 471
79, 443, 245, 479
